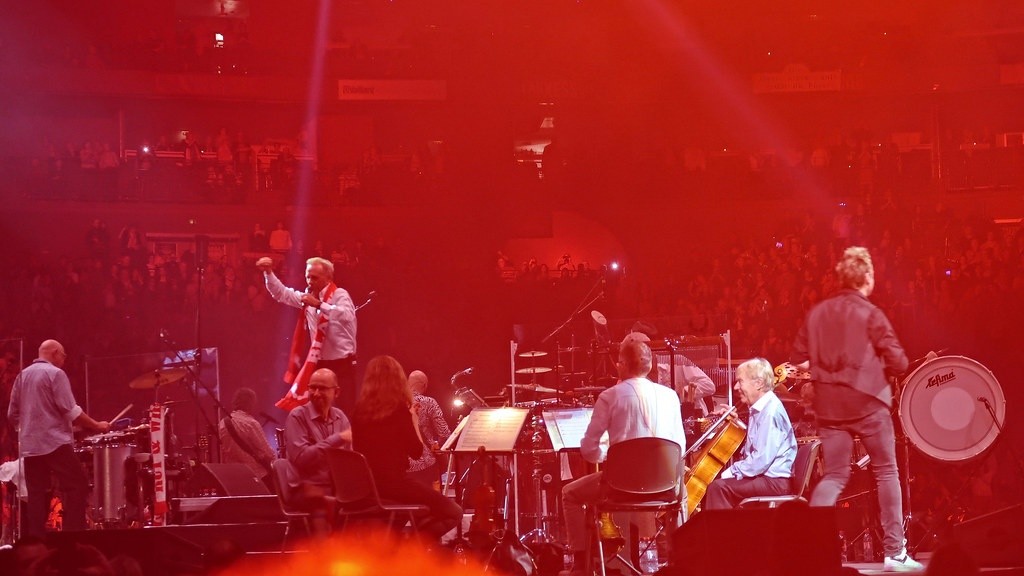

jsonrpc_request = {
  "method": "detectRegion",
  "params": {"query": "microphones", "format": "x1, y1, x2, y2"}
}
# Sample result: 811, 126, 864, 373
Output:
977, 396, 988, 402
160, 329, 164, 341
302, 286, 313, 307
602, 267, 606, 304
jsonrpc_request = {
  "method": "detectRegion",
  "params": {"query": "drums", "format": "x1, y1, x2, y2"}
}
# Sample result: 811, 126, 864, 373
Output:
898, 355, 1007, 463
87, 431, 139, 526
122, 424, 151, 454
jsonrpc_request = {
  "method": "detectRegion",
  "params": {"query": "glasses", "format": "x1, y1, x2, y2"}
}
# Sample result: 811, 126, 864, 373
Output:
306, 384, 338, 392
56, 347, 67, 359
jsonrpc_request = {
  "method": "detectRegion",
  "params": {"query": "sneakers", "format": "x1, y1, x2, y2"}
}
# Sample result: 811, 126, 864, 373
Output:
883, 538, 926, 573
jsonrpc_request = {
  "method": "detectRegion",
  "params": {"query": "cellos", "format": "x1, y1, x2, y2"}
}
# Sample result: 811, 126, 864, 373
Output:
679, 358, 789, 522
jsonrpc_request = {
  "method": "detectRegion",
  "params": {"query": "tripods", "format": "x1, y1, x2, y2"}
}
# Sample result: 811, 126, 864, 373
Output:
898, 436, 940, 561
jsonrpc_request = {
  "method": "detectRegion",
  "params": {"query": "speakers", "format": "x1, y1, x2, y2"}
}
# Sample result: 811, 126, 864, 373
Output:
182, 463, 271, 497
953, 501, 1024, 568
667, 505, 843, 576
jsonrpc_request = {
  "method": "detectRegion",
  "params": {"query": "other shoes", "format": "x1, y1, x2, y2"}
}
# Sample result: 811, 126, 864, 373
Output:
558, 551, 587, 576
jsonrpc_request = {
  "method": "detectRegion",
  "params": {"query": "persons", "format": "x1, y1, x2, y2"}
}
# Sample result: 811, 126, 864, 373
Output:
0, 524, 510, 576
406, 370, 451, 488
353, 355, 462, 543
7, 339, 110, 531
922, 544, 982, 576
218, 387, 275, 496
257, 257, 358, 414
705, 357, 797, 510
0, 117, 1024, 362
617, 321, 716, 418
793, 247, 925, 571
284, 368, 353, 518
558, 340, 687, 576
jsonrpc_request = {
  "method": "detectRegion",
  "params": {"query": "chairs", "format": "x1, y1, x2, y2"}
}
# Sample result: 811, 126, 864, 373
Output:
589, 437, 684, 576
202, 450, 429, 549
739, 441, 821, 505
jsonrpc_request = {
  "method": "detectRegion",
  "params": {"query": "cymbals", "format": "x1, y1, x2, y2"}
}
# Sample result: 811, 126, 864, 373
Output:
515, 366, 553, 374
127, 365, 196, 389
518, 351, 548, 358
505, 383, 565, 394
773, 390, 803, 401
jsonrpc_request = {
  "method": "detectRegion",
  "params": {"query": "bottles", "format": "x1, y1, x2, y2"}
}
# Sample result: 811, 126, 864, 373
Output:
839, 532, 848, 563
863, 529, 873, 562
639, 536, 660, 573
